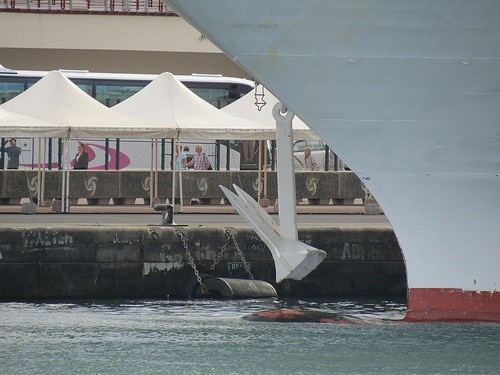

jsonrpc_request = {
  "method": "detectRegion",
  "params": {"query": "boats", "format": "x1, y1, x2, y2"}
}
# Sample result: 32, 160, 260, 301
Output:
161, 0, 498, 323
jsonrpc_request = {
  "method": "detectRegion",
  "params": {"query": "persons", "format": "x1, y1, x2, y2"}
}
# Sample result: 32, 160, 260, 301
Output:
74, 144, 88, 170
1, 138, 22, 169
243, 140, 255, 163
174, 144, 187, 171
183, 144, 211, 170
303, 147, 318, 171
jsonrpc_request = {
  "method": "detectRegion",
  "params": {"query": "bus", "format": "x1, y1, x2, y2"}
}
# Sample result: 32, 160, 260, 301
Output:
0, 63, 273, 171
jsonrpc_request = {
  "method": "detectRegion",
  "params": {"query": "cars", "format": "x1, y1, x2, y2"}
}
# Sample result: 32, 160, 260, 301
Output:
268, 137, 331, 170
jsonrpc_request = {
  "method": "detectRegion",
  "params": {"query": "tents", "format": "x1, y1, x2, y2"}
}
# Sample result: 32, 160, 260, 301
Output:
0, 70, 112, 213
80, 71, 256, 209
223, 80, 342, 205
